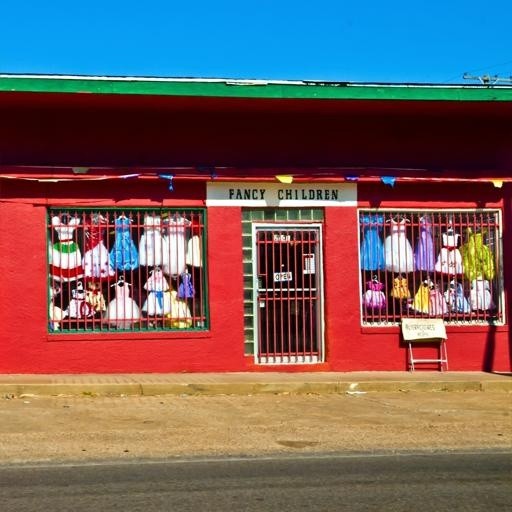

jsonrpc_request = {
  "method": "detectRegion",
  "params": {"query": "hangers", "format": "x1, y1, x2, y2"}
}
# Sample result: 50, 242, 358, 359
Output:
359, 208, 495, 292
48, 209, 202, 301
